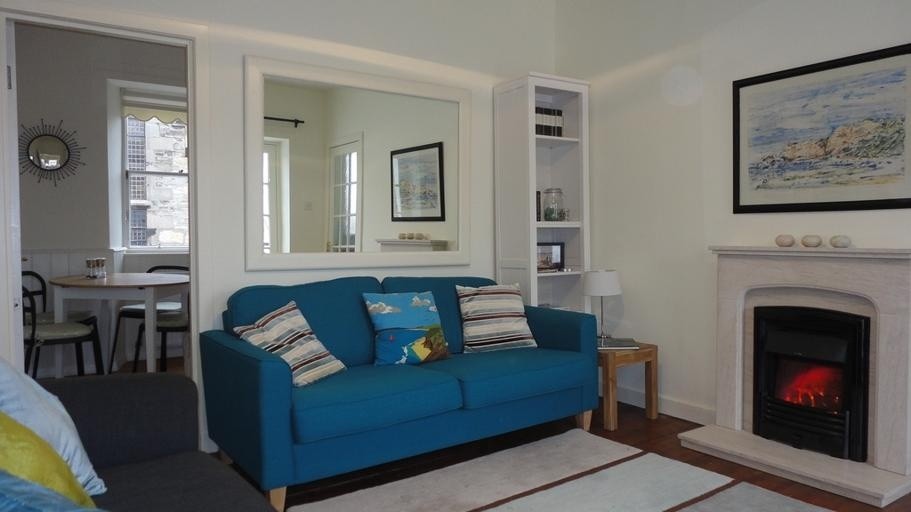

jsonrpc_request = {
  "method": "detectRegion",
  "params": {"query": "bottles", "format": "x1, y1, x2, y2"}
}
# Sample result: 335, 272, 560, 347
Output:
94, 258, 106, 279
85, 258, 95, 279
541, 187, 565, 221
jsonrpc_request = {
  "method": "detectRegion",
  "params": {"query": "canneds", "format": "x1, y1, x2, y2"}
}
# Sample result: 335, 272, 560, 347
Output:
85, 258, 95, 277
95, 256, 108, 277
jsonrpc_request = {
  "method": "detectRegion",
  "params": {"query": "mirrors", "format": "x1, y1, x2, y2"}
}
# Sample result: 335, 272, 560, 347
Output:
18, 117, 86, 185
241, 54, 472, 273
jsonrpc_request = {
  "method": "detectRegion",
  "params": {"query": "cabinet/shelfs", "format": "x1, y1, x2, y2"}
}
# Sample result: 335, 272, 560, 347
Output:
491, 72, 592, 317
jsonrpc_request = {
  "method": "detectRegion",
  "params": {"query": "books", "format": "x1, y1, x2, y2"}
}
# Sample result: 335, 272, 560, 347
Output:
596, 337, 640, 350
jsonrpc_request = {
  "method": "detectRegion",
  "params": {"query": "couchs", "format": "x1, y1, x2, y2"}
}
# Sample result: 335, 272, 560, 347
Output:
196, 274, 599, 512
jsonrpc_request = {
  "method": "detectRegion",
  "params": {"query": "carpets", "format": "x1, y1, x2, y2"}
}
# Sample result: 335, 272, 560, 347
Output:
284, 427, 831, 512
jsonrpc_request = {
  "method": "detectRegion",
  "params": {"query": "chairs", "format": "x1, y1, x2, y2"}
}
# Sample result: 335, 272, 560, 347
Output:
108, 265, 191, 374
133, 289, 191, 375
20, 270, 103, 374
21, 286, 103, 377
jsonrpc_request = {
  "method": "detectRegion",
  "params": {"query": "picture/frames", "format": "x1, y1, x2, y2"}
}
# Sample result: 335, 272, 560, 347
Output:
731, 44, 910, 216
536, 242, 565, 272
389, 142, 446, 223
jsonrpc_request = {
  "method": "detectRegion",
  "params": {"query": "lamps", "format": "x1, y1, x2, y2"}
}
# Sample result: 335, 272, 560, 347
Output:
580, 269, 622, 345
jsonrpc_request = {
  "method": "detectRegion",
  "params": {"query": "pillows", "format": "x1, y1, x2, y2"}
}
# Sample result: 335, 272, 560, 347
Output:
360, 290, 450, 368
0, 410, 96, 508
230, 299, 348, 388
0, 468, 106, 512
0, 355, 107, 497
26, 371, 278, 512
454, 283, 538, 354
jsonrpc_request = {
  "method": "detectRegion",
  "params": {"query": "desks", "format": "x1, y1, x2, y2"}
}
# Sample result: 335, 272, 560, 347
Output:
594, 342, 658, 432
48, 271, 189, 379
